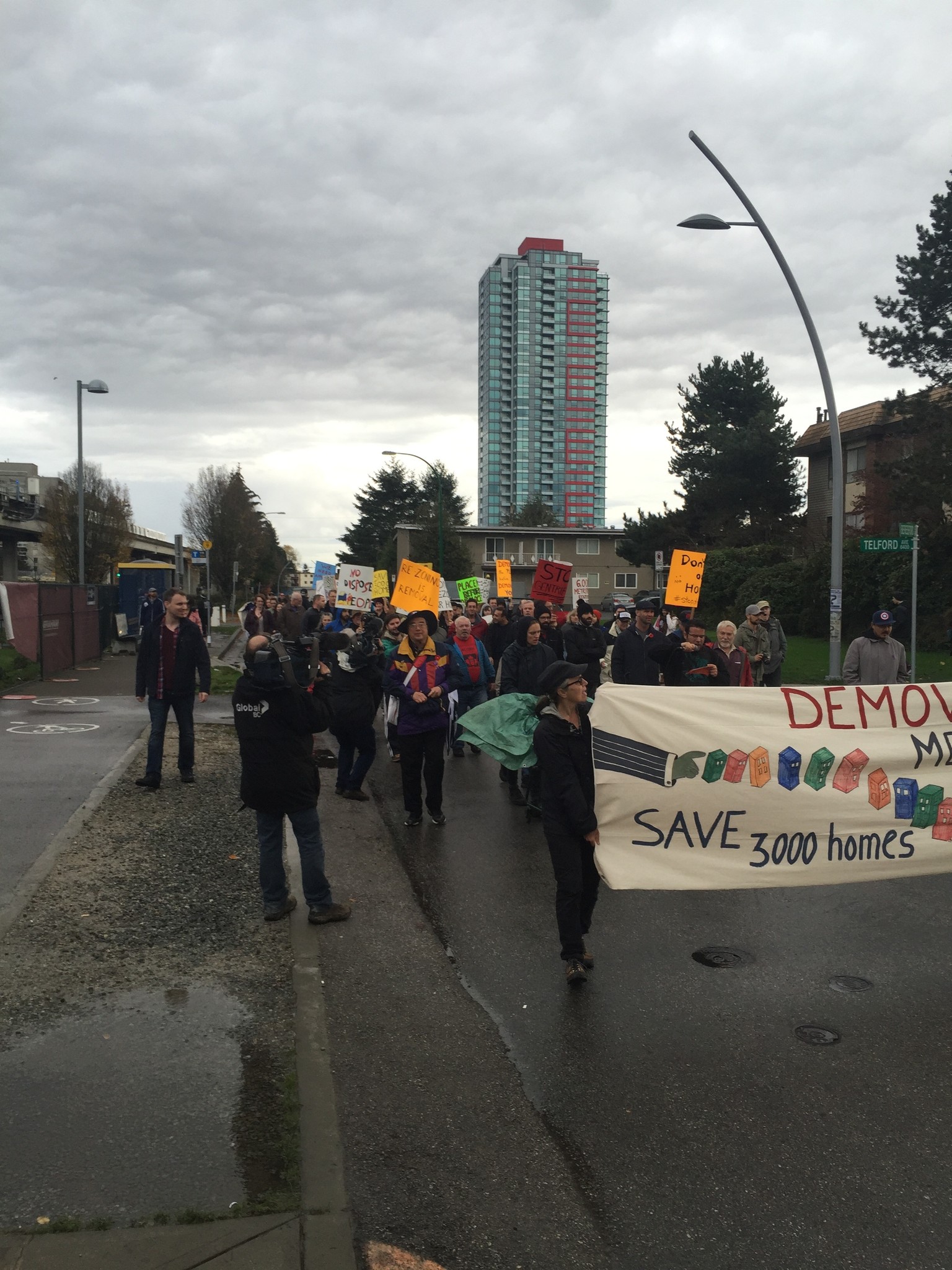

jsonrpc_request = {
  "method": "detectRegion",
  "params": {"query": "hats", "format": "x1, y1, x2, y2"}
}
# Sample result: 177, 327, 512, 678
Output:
397, 610, 438, 636
745, 600, 769, 616
149, 587, 157, 592
384, 613, 400, 626
375, 597, 385, 606
496, 598, 697, 627
892, 591, 905, 601
872, 610, 897, 625
538, 659, 589, 695
300, 588, 307, 594
352, 610, 364, 617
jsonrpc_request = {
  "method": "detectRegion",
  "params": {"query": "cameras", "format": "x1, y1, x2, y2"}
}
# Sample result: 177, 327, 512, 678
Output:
756, 655, 771, 665
255, 630, 328, 684
355, 613, 380, 655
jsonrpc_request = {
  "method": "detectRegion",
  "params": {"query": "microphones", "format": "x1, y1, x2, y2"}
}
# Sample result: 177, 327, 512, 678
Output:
676, 645, 698, 652
317, 632, 350, 650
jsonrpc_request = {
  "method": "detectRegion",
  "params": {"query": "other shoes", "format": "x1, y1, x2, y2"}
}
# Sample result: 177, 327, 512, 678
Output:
179, 766, 194, 783
136, 775, 160, 788
335, 786, 370, 801
452, 743, 481, 758
508, 783, 527, 805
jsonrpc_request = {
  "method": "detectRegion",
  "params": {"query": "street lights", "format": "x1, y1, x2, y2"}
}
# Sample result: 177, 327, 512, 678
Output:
674, 132, 846, 688
379, 450, 445, 578
76, 377, 110, 582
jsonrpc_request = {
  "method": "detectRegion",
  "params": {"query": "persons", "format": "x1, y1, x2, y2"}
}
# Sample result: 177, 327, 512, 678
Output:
330, 629, 386, 803
598, 610, 632, 684
611, 599, 672, 687
651, 619, 730, 687
889, 594, 911, 674
565, 599, 607, 697
500, 616, 558, 806
533, 660, 600, 984
655, 604, 678, 636
378, 616, 408, 762
706, 620, 753, 687
446, 614, 496, 758
733, 603, 771, 686
756, 600, 788, 687
135, 586, 211, 788
231, 634, 355, 923
841, 609, 910, 686
184, 598, 203, 640
383, 611, 462, 826
197, 593, 213, 636
139, 587, 166, 636
605, 603, 628, 629
665, 610, 710, 645
236, 588, 607, 659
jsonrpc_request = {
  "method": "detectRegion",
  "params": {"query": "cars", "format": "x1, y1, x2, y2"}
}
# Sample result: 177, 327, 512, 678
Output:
601, 592, 635, 612
633, 590, 664, 609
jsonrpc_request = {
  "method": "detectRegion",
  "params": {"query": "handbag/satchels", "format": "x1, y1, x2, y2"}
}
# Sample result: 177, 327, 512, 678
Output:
387, 696, 401, 725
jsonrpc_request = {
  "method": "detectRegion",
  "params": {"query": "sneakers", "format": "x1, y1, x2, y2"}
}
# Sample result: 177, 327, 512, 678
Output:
263, 894, 298, 922
566, 936, 594, 985
403, 807, 447, 824
308, 902, 352, 925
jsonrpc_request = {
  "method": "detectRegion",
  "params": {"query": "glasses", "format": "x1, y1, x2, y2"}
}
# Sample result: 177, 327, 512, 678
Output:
562, 675, 582, 690
688, 633, 706, 639
407, 623, 428, 629
330, 595, 336, 598
